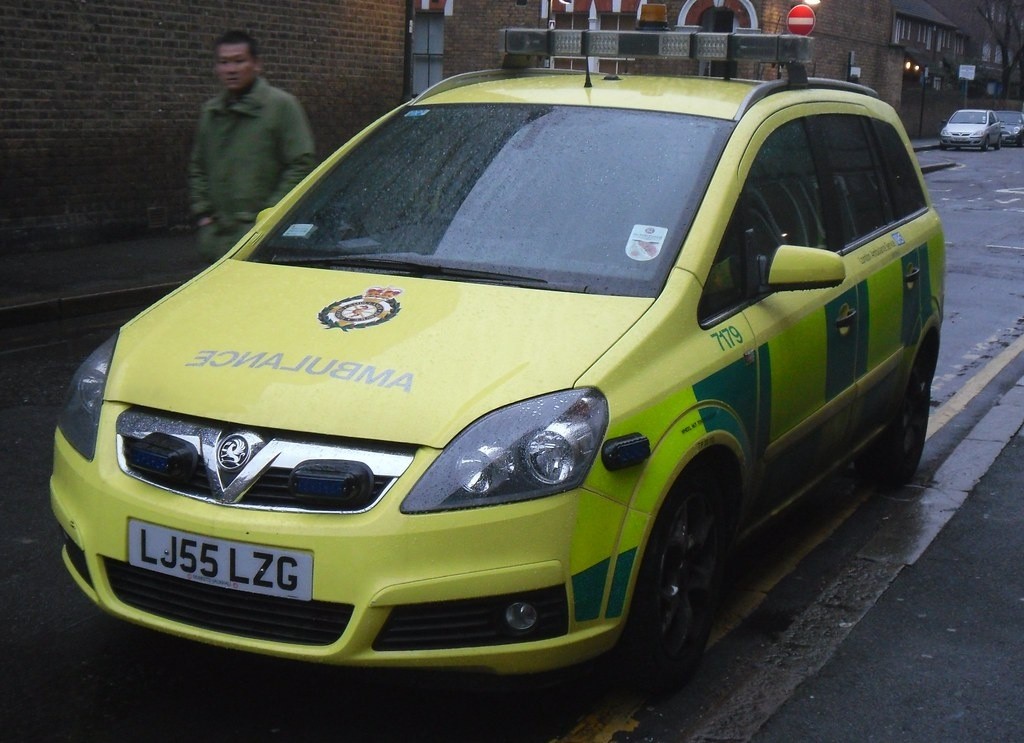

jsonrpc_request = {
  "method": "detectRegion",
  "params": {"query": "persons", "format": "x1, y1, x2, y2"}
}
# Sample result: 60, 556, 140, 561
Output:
188, 29, 315, 270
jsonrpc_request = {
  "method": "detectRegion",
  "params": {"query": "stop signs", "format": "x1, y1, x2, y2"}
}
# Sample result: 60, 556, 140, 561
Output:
786, 4, 816, 36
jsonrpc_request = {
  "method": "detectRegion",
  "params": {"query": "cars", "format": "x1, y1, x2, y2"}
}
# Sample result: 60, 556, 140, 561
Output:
996, 110, 1024, 147
938, 108, 1002, 152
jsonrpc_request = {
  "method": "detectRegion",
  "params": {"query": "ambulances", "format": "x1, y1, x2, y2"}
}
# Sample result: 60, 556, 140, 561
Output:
46, 26, 948, 691
